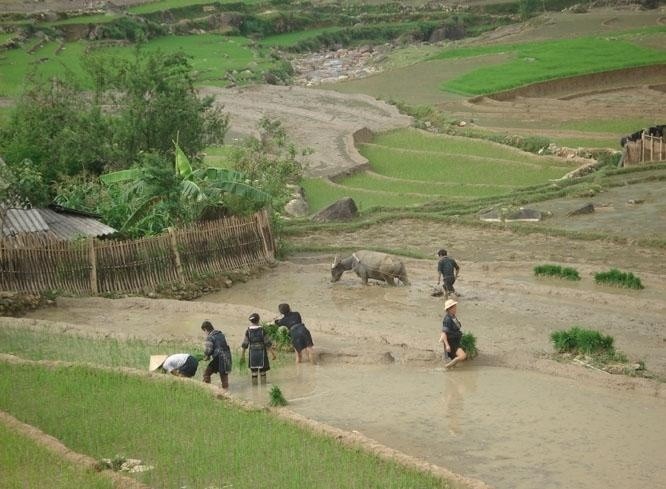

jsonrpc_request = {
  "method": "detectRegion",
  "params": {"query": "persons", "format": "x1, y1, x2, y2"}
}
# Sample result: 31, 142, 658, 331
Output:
238, 313, 273, 388
199, 321, 231, 389
440, 299, 467, 370
147, 353, 197, 379
274, 303, 317, 366
437, 249, 461, 301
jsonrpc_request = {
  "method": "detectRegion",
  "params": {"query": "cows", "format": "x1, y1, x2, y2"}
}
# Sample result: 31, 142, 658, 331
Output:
621, 125, 666, 147
331, 250, 412, 288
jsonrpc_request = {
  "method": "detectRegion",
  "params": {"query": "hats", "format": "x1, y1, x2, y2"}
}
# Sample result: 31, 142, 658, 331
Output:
444, 299, 458, 311
149, 355, 168, 372
